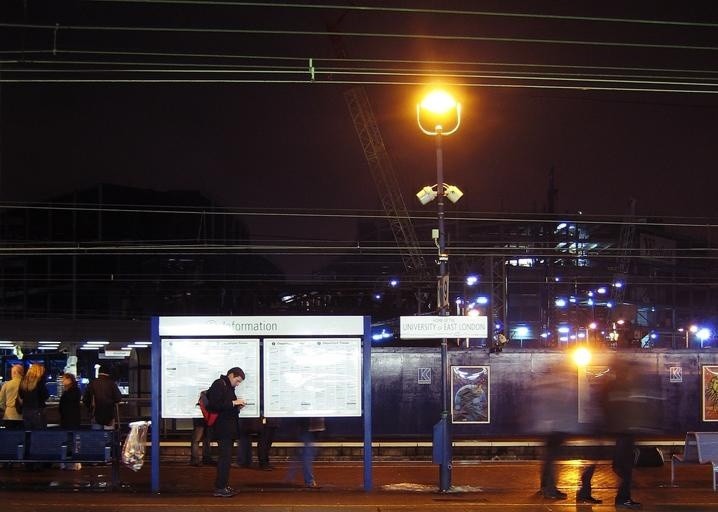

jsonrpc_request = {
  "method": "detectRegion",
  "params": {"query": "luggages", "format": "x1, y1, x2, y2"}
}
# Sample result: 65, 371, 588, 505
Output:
80, 405, 96, 426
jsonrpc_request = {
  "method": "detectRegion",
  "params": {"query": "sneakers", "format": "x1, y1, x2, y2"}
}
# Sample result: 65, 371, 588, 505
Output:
189, 458, 275, 470
226, 486, 240, 495
0, 462, 113, 471
304, 481, 321, 488
540, 491, 642, 508
214, 487, 234, 497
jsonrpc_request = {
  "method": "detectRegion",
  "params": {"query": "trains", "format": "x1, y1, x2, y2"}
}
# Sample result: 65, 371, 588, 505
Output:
309, 333, 718, 447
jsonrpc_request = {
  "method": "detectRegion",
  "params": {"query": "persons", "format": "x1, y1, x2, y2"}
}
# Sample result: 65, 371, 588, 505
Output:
0, 364, 27, 471
197, 367, 246, 498
19, 362, 50, 429
84, 366, 122, 466
536, 433, 640, 508
190, 417, 326, 489
52, 375, 82, 471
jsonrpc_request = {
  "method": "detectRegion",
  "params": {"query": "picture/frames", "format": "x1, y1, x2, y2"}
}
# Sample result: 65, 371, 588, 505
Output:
701, 364, 718, 422
450, 364, 490, 425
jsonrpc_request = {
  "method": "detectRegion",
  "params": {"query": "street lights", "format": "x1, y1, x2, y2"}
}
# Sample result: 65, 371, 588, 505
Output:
698, 327, 710, 347
585, 322, 597, 342
684, 324, 697, 347
411, 89, 464, 489
612, 320, 626, 343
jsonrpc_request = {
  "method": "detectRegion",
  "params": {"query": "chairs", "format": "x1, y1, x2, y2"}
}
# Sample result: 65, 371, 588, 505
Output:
1, 424, 121, 495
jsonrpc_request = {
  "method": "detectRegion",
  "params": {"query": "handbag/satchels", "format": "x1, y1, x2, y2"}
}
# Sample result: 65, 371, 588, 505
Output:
198, 379, 228, 427
15, 395, 23, 414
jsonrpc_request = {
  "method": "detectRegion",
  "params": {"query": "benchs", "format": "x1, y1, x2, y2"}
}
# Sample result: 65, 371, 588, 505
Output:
691, 431, 718, 492
666, 429, 712, 489
